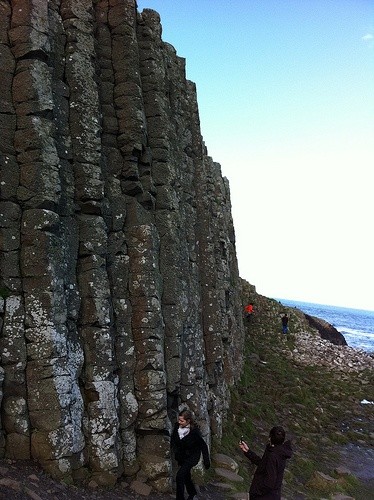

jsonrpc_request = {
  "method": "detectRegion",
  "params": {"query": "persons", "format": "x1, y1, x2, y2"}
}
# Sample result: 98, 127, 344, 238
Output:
237, 426, 293, 500
168, 410, 211, 500
244, 302, 255, 321
282, 311, 290, 335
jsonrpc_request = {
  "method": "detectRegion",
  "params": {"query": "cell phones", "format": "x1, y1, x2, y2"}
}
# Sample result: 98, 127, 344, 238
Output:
240, 435, 244, 445
